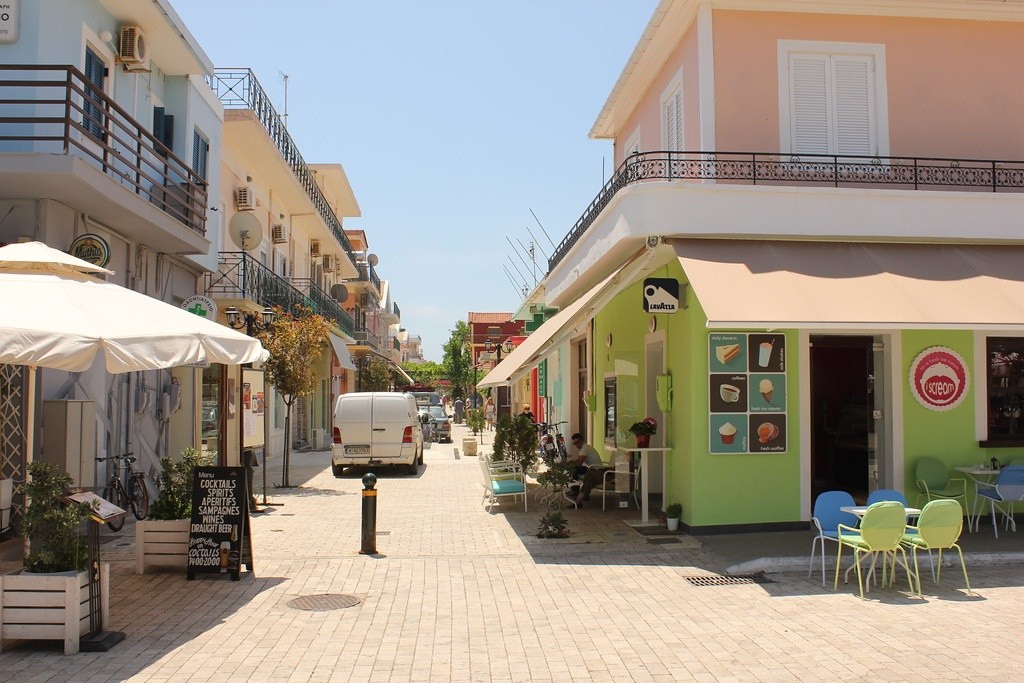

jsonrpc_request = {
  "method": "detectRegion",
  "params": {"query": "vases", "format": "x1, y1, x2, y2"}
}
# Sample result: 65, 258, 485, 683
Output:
636, 434, 649, 448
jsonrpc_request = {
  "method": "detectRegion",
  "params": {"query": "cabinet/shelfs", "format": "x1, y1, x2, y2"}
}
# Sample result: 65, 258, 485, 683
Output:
202, 383, 219, 440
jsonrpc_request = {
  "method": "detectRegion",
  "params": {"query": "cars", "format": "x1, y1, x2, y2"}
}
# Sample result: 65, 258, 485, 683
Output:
417, 405, 452, 443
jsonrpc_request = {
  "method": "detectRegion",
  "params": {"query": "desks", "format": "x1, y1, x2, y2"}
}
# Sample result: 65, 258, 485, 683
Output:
618, 445, 672, 527
526, 473, 582, 510
840, 505, 922, 587
954, 466, 1002, 531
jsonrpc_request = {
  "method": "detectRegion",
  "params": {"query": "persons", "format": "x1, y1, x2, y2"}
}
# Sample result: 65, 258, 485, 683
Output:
485, 398, 496, 431
519, 404, 536, 455
561, 433, 603, 509
442, 395, 471, 423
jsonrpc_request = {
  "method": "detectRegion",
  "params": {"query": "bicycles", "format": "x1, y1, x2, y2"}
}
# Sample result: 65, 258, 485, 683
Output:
95, 452, 149, 532
420, 413, 440, 443
549, 420, 569, 462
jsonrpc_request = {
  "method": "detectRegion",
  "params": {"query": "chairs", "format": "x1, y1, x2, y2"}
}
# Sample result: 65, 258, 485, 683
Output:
478, 452, 642, 514
808, 458, 1024, 599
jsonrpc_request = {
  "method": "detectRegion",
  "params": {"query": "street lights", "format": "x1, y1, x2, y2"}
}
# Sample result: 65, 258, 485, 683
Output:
485, 336, 514, 431
223, 303, 279, 512
474, 360, 487, 436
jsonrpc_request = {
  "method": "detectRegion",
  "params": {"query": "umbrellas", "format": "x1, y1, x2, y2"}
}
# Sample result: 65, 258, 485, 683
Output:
0, 242, 270, 557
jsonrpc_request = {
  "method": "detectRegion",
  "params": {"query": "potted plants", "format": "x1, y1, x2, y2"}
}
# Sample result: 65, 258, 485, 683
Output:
135, 446, 215, 575
664, 503, 683, 531
0, 457, 110, 655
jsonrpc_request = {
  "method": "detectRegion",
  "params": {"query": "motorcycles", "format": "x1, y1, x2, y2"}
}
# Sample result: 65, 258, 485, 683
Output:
534, 422, 558, 465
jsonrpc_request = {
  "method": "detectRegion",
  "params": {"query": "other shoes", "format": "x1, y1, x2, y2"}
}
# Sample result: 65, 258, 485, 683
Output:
567, 502, 584, 508
583, 496, 590, 500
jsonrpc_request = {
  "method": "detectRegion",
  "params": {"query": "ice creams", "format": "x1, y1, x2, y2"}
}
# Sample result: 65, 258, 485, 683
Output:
759, 379, 773, 403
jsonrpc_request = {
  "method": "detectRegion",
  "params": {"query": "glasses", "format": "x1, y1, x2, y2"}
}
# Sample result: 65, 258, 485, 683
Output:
573, 440, 579, 445
524, 407, 529, 410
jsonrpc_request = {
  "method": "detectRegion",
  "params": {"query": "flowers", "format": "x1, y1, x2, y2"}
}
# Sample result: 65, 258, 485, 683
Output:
629, 417, 657, 436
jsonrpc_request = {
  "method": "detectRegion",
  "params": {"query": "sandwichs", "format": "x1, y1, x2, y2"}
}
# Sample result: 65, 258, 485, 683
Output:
716, 344, 740, 364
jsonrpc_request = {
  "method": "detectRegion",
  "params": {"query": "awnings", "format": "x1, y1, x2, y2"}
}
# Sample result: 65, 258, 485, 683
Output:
476, 250, 650, 388
370, 350, 396, 366
672, 240, 1024, 329
329, 332, 357, 371
394, 364, 414, 386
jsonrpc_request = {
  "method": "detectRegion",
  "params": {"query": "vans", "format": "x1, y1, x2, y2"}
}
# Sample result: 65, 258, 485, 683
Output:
412, 392, 442, 408
331, 390, 424, 478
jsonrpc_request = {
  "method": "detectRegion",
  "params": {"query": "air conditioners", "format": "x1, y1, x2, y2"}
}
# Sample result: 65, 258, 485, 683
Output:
362, 293, 371, 309
313, 241, 322, 257
120, 25, 152, 71
323, 254, 334, 272
238, 187, 257, 210
271, 224, 288, 241
